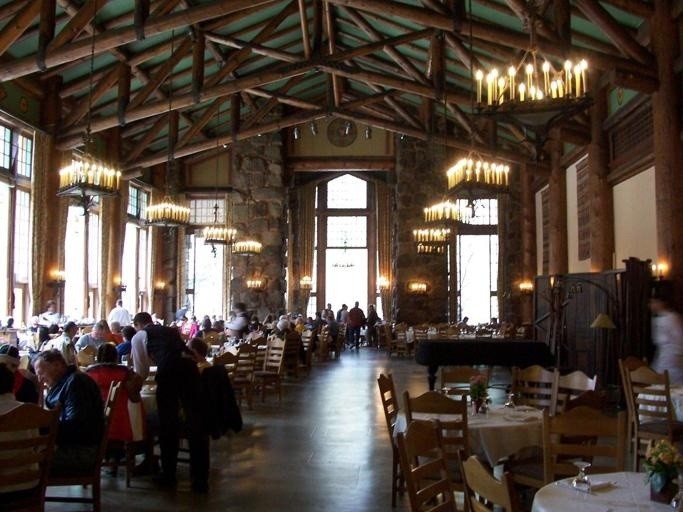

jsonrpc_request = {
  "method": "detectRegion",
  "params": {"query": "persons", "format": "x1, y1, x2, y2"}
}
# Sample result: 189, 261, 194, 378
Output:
25, 299, 135, 367
337, 304, 346, 322
133, 312, 210, 496
1, 364, 44, 512
5, 318, 20, 346
648, 291, 683, 386
159, 303, 335, 369
348, 302, 365, 349
84, 344, 152, 477
0, 344, 38, 402
341, 306, 348, 323
458, 318, 468, 326
32, 348, 105, 478
366, 304, 379, 346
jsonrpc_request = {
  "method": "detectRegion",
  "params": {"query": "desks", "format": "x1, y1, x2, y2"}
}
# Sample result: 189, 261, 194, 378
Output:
417, 335, 552, 390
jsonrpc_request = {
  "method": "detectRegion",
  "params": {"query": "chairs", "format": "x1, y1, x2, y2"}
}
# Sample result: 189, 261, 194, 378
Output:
384, 325, 406, 359
300, 330, 315, 369
322, 324, 334, 355
413, 326, 429, 357
212, 351, 243, 388
252, 338, 287, 403
236, 342, 260, 410
338, 322, 347, 356
35, 380, 125, 511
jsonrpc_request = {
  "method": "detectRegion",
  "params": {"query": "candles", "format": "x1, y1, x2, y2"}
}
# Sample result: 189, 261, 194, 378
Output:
248, 280, 261, 288
653, 263, 665, 280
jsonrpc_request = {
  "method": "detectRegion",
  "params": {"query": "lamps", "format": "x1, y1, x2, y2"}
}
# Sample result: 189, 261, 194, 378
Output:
232, 235, 262, 257
55, 0, 123, 190
293, 120, 373, 139
300, 275, 313, 298
409, 282, 427, 296
144, 28, 192, 222
423, 199, 461, 225
537, 274, 616, 370
0, 400, 64, 511
473, 21, 596, 107
203, 100, 238, 245
445, 97, 512, 197
411, 226, 450, 256
519, 279, 534, 295
376, 276, 389, 293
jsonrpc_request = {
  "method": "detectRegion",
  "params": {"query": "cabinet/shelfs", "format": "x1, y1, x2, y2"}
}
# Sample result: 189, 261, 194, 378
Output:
530, 270, 650, 378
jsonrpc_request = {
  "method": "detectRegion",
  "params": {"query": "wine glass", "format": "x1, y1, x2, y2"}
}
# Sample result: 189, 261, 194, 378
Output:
441, 387, 516, 418
670, 479, 683, 511
459, 327, 502, 336
569, 461, 592, 493
121, 355, 127, 365
